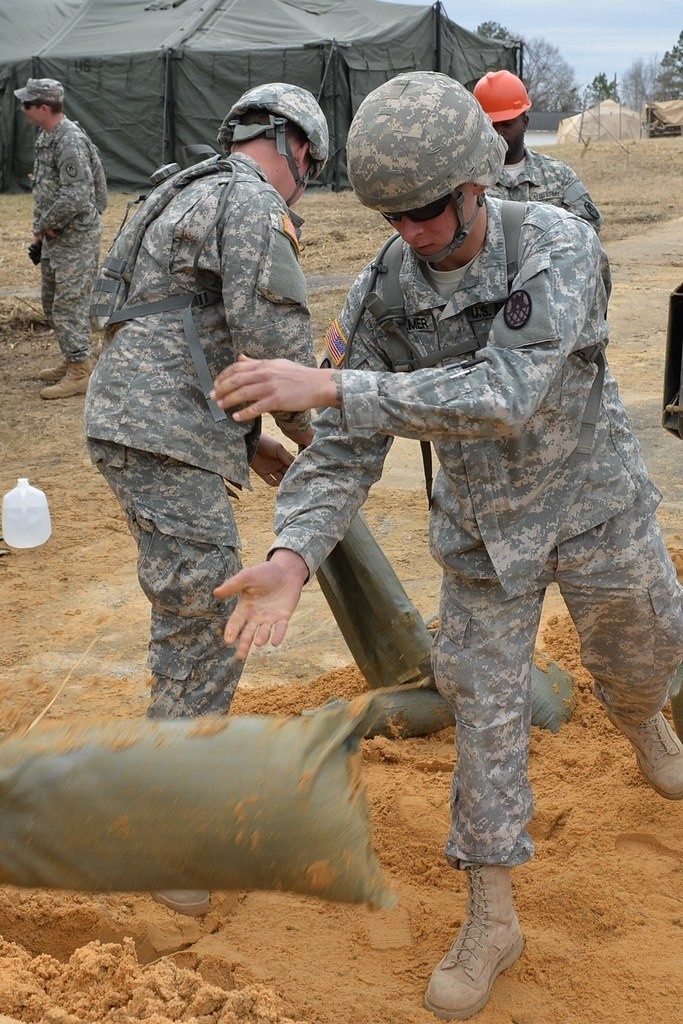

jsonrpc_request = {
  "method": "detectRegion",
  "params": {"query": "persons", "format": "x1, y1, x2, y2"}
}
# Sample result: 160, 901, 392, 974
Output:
473, 71, 601, 238
83, 82, 330, 723
13, 76, 108, 401
209, 71, 682, 1021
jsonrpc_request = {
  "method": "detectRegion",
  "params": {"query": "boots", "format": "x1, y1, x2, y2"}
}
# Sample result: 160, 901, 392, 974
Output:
39, 358, 94, 380
607, 702, 683, 801
423, 861, 525, 1020
40, 359, 93, 400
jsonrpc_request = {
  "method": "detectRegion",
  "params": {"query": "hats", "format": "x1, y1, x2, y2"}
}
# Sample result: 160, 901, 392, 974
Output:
14, 77, 66, 104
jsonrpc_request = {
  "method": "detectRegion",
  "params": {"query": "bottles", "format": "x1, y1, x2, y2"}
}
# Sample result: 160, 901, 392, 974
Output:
1, 477, 52, 550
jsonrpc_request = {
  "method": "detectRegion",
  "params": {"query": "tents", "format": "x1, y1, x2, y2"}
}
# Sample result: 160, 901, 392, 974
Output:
0, 0, 527, 194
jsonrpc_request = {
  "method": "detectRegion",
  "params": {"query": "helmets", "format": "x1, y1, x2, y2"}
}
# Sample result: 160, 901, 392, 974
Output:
216, 83, 328, 183
474, 70, 532, 123
347, 69, 509, 211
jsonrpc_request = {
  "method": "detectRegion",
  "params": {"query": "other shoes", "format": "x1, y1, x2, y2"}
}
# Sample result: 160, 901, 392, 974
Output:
33, 323, 54, 336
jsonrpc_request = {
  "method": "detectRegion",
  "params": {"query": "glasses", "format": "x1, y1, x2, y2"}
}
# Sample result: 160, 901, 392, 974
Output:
18, 99, 40, 110
380, 194, 451, 222
